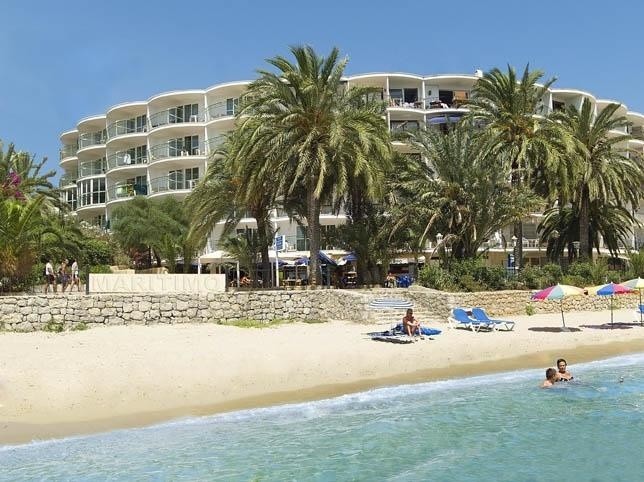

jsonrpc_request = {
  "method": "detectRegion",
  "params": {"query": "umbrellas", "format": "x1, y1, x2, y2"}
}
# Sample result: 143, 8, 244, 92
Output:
587, 281, 636, 324
529, 282, 585, 328
616, 277, 644, 324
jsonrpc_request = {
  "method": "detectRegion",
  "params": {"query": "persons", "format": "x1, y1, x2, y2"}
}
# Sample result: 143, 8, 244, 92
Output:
68, 259, 83, 293
60, 258, 70, 292
402, 308, 423, 341
554, 360, 573, 383
539, 368, 557, 389
387, 272, 397, 289
43, 259, 57, 292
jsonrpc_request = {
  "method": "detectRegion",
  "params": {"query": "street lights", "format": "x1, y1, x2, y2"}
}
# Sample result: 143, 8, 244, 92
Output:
572, 241, 581, 263
511, 235, 519, 290
436, 233, 444, 290
550, 229, 560, 264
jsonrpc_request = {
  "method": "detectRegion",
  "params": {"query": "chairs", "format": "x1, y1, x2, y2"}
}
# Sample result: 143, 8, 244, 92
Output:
448, 307, 516, 334
